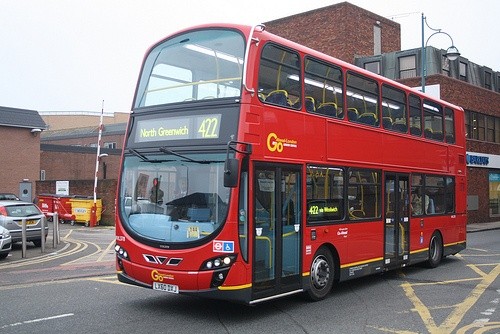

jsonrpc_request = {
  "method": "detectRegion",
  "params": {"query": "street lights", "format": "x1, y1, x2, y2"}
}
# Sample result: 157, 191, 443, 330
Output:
421, 11, 461, 94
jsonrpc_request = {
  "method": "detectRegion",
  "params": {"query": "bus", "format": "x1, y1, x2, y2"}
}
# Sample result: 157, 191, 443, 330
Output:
113, 21, 470, 306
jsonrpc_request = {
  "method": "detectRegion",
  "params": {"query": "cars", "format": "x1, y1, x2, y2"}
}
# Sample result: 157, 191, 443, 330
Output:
0, 192, 49, 248
0, 225, 12, 261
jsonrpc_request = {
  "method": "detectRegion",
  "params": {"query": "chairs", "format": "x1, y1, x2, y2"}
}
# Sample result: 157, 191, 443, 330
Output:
427, 199, 435, 214
258, 90, 454, 143
184, 96, 215, 102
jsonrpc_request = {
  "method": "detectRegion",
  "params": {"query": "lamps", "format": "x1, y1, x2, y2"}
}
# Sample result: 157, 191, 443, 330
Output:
30, 128, 41, 137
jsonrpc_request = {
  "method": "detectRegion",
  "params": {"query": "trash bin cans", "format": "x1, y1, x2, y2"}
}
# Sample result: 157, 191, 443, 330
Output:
37, 193, 87, 226
70, 197, 102, 227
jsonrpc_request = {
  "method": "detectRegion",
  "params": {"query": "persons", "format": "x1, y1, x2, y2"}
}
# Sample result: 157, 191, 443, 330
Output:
175, 176, 196, 220
412, 186, 429, 216
